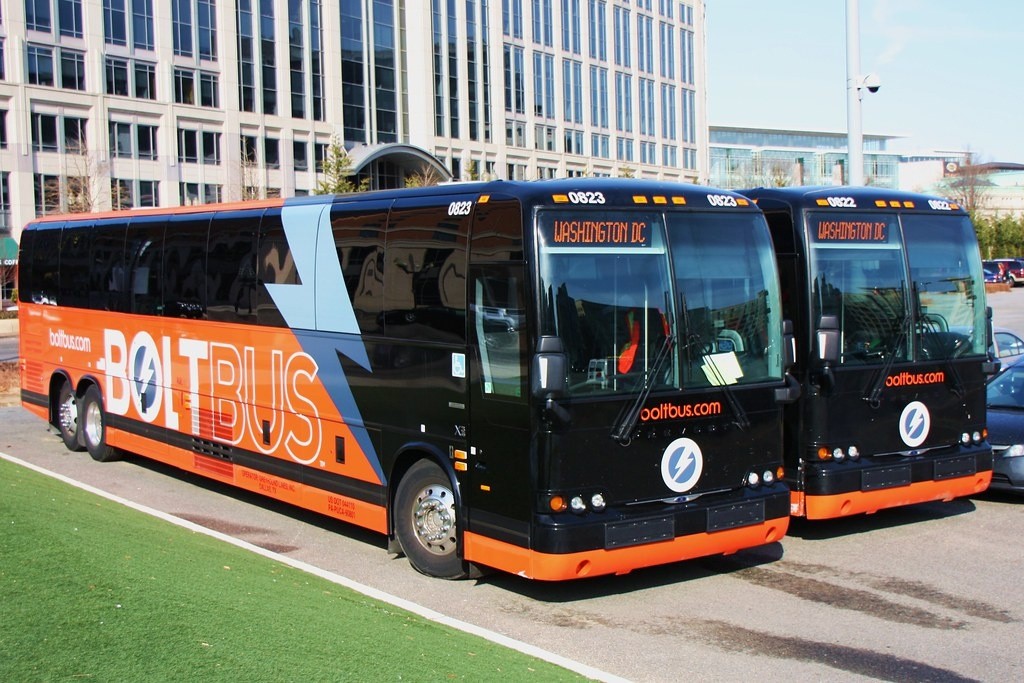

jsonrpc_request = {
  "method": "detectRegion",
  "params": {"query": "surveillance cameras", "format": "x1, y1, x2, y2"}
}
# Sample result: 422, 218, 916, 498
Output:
866, 73, 881, 93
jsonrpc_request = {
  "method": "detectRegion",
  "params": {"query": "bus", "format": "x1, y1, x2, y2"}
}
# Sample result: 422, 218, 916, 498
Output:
730, 184, 1003, 521
16, 178, 797, 584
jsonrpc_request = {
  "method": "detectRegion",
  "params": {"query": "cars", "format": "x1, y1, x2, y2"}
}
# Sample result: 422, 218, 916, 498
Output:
982, 257, 1024, 287
916, 325, 1024, 379
988, 355, 1024, 489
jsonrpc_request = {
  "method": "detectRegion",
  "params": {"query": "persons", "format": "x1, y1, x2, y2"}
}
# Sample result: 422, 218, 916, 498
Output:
998, 263, 1005, 279
